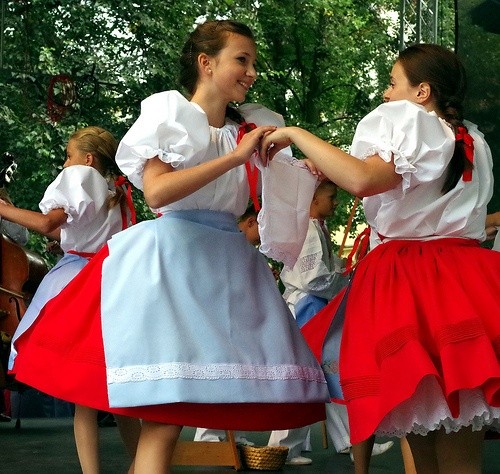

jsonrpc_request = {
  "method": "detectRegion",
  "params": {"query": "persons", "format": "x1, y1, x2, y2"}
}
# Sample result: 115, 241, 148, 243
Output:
194, 176, 500, 466
0, 127, 141, 474
259, 44, 500, 474
14, 18, 332, 474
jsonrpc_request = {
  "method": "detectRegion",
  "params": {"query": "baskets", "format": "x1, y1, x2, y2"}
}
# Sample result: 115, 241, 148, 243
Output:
240, 443, 292, 473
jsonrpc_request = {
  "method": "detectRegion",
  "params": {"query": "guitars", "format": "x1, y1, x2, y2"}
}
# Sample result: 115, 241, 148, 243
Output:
0, 232, 29, 387
